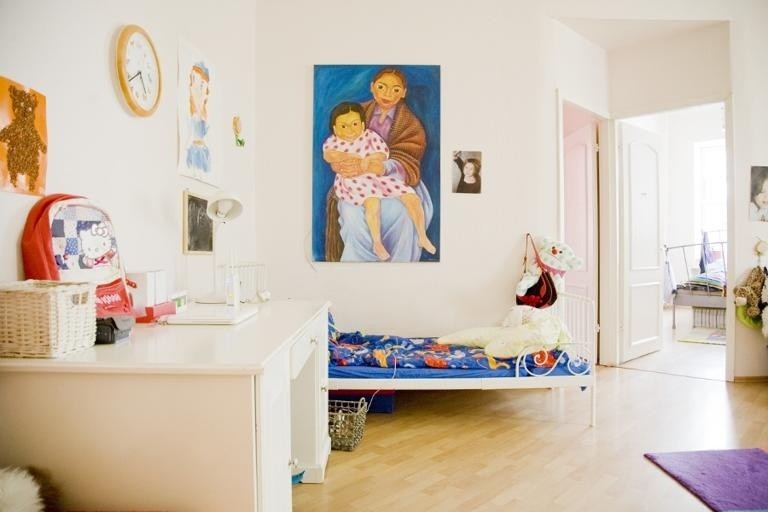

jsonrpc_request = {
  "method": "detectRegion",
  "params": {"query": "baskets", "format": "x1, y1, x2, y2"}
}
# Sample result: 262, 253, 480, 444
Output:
0, 280, 97, 359
328, 397, 368, 451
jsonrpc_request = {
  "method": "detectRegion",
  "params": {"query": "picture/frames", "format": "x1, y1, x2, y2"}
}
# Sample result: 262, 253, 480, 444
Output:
183, 191, 216, 254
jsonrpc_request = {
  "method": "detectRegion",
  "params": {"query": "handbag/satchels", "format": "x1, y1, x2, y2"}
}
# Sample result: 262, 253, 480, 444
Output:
516, 233, 557, 309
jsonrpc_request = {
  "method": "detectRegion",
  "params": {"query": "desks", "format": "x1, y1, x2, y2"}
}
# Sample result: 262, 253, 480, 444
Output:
0, 299, 331, 512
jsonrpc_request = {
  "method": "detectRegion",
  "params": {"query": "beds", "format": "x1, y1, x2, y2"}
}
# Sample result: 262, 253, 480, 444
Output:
663, 241, 727, 330
331, 324, 596, 428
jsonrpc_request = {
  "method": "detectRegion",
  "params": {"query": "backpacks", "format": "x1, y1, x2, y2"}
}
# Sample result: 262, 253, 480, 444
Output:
21, 193, 136, 320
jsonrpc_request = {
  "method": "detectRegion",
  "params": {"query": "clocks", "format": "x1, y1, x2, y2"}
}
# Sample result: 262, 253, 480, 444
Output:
116, 24, 163, 116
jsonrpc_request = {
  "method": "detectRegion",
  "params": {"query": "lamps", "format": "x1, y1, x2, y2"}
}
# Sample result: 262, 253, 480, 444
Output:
192, 189, 242, 305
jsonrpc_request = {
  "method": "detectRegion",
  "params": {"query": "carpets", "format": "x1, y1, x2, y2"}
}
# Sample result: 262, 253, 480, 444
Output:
643, 448, 768, 512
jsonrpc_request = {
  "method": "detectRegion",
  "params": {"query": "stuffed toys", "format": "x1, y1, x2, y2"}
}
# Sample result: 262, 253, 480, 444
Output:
732, 266, 768, 338
515, 236, 585, 311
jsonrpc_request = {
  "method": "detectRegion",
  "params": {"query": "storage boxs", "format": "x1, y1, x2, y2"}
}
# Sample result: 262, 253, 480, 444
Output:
328, 389, 395, 415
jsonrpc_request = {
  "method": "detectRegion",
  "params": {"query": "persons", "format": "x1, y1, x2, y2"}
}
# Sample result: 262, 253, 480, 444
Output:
321, 66, 427, 262
321, 99, 437, 261
452, 150, 481, 195
750, 168, 768, 222
182, 61, 215, 174
